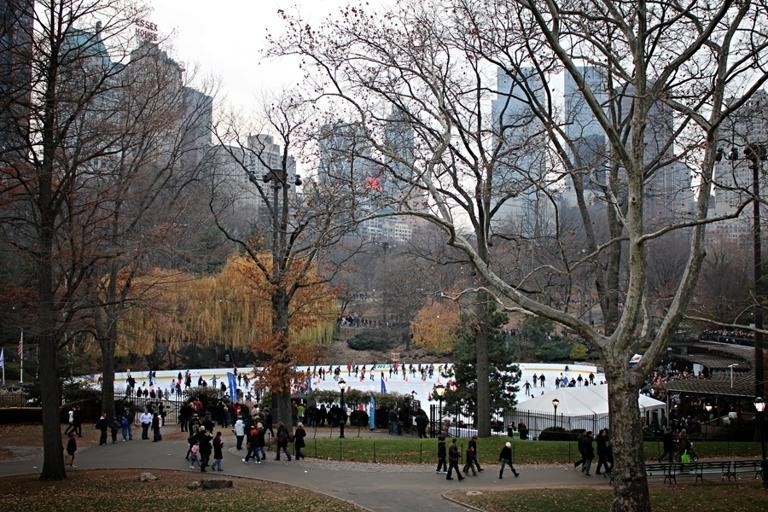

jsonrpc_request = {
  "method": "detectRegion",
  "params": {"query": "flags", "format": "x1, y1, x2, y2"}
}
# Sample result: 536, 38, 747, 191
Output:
16, 330, 23, 358
0, 347, 4, 368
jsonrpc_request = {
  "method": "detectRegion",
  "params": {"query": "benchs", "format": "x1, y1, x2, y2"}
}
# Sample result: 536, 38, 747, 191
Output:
732, 460, 764, 482
609, 459, 672, 488
671, 461, 731, 483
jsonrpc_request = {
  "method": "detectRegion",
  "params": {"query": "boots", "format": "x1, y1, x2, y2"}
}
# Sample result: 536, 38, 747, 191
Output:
498, 469, 503, 479
511, 468, 520, 478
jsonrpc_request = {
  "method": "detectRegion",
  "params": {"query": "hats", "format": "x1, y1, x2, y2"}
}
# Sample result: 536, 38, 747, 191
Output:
505, 441, 512, 448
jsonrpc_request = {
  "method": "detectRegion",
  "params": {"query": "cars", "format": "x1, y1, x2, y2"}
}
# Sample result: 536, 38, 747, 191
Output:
0, 383, 42, 408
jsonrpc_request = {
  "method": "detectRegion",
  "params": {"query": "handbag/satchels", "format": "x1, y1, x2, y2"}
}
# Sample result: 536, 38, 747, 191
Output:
680, 450, 691, 463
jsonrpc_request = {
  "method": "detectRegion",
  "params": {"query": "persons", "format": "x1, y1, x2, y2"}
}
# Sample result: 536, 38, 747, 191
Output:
65, 407, 79, 434
66, 432, 76, 466
68, 406, 81, 437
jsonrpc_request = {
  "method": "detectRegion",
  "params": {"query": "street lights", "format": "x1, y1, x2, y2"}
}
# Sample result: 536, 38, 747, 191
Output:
338, 378, 345, 409
238, 165, 307, 279
751, 393, 767, 489
703, 402, 712, 442
380, 241, 390, 323
727, 362, 739, 390
551, 399, 559, 427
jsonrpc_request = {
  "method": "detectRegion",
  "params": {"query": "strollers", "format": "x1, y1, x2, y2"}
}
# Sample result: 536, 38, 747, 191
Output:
673, 441, 699, 463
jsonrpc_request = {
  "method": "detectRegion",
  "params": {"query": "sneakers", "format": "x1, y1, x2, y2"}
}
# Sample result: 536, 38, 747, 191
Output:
458, 476, 466, 481
478, 468, 484, 472
445, 477, 454, 481
472, 474, 477, 477
463, 470, 466, 473
64, 429, 311, 474
435, 470, 441, 474
465, 473, 470, 476
442, 470, 448, 473
658, 458, 674, 462
574, 461, 613, 476
